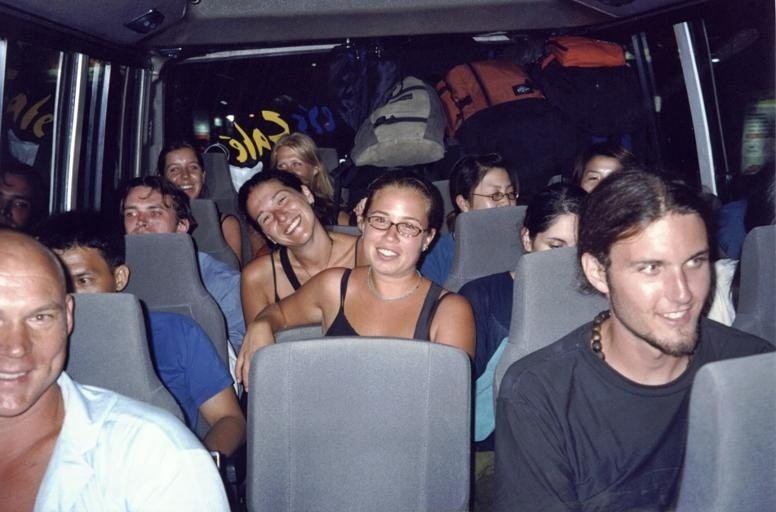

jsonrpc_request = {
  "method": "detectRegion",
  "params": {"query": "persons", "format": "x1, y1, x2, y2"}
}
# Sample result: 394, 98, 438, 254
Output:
2, 73, 776, 512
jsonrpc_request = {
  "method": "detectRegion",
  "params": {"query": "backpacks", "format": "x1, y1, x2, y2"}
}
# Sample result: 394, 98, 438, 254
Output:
166, 36, 402, 168
434, 57, 580, 185
349, 75, 449, 168
517, 30, 653, 138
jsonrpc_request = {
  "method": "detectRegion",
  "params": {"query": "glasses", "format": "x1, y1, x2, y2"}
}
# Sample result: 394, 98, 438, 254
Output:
472, 190, 519, 202
362, 215, 429, 238
